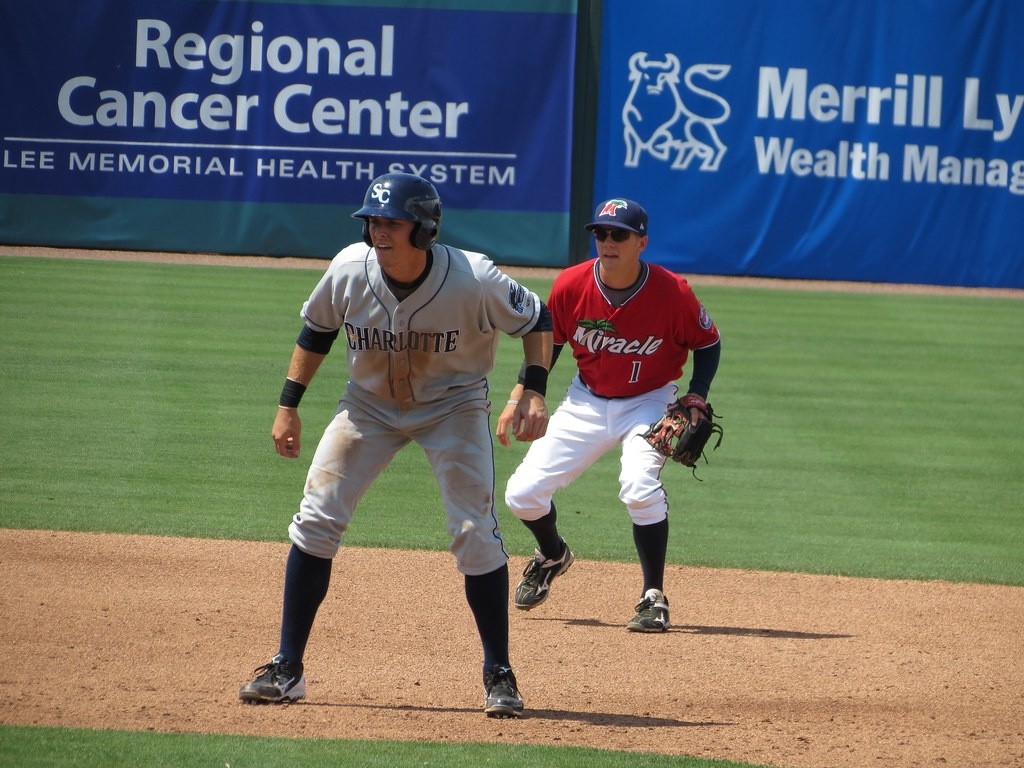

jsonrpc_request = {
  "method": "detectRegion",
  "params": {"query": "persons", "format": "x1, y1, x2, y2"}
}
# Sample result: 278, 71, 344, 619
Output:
496, 198, 722, 633
240, 172, 553, 719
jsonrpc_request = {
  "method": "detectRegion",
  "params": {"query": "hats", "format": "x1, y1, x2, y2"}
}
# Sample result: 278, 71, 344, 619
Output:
584, 198, 648, 235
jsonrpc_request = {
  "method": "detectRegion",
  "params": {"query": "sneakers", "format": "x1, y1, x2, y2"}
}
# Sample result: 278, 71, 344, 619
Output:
515, 536, 574, 611
626, 588, 671, 633
482, 665, 523, 717
239, 653, 306, 703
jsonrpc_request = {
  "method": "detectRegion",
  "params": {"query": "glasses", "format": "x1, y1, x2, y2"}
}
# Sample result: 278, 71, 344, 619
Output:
594, 229, 631, 242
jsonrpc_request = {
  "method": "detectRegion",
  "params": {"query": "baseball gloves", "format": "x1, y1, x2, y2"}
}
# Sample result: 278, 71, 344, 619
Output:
641, 394, 715, 468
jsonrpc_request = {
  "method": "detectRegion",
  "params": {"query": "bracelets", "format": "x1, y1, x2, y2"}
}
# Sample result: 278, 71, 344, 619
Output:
277, 377, 307, 410
506, 400, 518, 405
523, 365, 549, 398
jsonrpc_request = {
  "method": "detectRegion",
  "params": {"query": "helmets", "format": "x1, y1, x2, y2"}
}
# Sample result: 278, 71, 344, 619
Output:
351, 174, 441, 250
